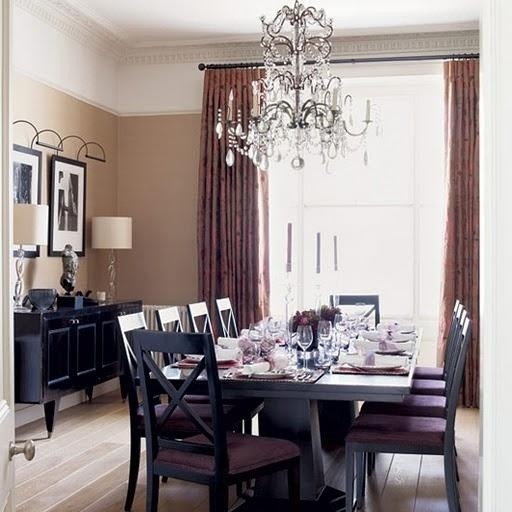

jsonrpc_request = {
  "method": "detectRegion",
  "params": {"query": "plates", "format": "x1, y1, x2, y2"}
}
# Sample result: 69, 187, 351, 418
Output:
251, 372, 293, 379
353, 331, 411, 369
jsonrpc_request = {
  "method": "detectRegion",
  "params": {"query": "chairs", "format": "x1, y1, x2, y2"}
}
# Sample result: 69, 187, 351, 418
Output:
117, 294, 300, 511
336, 296, 380, 325
345, 298, 474, 510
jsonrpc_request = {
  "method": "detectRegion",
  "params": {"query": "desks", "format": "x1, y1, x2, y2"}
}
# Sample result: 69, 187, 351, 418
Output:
137, 319, 420, 511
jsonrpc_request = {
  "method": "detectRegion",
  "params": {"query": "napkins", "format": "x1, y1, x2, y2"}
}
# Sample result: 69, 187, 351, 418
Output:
353, 321, 403, 369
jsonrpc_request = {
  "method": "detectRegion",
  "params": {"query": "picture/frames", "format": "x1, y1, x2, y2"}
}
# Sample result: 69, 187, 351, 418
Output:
49, 155, 87, 257
11, 144, 43, 259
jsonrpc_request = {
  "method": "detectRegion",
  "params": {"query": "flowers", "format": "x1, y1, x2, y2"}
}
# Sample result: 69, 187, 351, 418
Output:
289, 305, 342, 353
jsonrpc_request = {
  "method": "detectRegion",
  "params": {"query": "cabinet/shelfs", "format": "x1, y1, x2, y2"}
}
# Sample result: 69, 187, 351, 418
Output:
13, 299, 143, 440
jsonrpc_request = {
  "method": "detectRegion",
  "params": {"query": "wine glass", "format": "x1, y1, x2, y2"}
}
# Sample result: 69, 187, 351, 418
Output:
334, 314, 349, 352
354, 302, 365, 323
296, 325, 314, 370
347, 320, 359, 343
317, 321, 332, 359
248, 323, 264, 358
267, 317, 281, 344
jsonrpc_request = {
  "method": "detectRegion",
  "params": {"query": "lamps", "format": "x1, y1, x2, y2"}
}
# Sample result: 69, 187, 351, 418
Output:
90, 216, 133, 299
12, 203, 49, 313
214, 0, 383, 176
